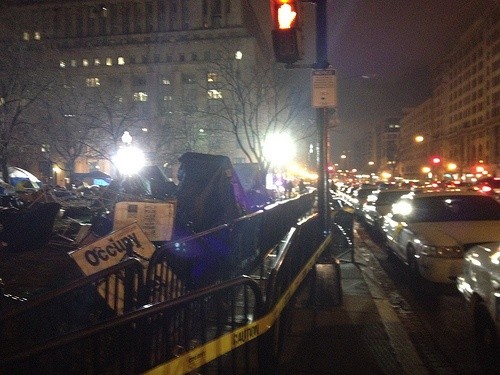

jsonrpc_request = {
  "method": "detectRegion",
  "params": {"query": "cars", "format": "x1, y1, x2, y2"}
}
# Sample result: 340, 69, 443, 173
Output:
364, 189, 422, 234
382, 191, 500, 285
333, 177, 500, 220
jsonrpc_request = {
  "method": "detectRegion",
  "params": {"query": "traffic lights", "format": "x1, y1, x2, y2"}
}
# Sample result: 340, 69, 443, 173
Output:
270, 0, 302, 63
